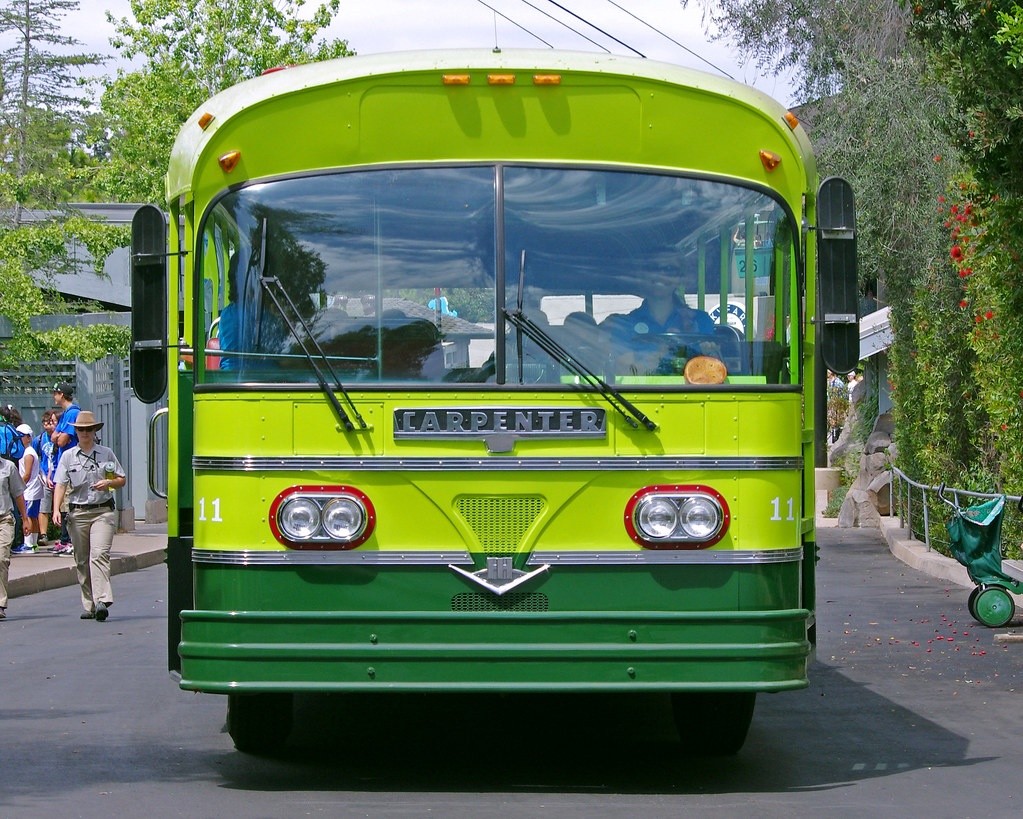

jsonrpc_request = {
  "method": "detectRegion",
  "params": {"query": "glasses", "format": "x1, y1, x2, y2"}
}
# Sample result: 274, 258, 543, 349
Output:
75, 427, 95, 431
19, 433, 25, 437
645, 262, 682, 278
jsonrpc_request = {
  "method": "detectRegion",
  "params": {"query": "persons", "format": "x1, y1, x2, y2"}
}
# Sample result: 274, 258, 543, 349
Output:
826, 369, 863, 403
600, 252, 721, 376
219, 247, 292, 369
0, 457, 31, 618
51, 381, 81, 557
0, 405, 70, 553
52, 411, 126, 620
560, 313, 599, 347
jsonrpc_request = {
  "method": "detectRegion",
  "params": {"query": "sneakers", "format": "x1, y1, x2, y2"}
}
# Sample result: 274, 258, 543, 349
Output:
81, 610, 96, 619
34, 545, 39, 552
37, 534, 48, 545
11, 543, 35, 554
95, 602, 108, 621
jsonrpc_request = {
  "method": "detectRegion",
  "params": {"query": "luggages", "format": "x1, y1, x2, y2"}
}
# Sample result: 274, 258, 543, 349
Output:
938, 482, 1023, 627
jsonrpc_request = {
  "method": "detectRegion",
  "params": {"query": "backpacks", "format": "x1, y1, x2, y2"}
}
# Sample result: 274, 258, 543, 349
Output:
0, 422, 25, 459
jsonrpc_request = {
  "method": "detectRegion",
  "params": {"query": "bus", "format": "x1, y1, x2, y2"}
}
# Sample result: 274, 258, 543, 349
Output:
131, 46, 862, 757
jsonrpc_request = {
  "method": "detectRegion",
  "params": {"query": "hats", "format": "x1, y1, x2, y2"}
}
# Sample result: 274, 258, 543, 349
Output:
66, 411, 104, 431
847, 371, 856, 377
53, 382, 73, 396
16, 424, 32, 438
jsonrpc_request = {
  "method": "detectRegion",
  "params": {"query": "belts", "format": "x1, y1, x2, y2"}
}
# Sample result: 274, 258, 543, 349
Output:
69, 498, 114, 508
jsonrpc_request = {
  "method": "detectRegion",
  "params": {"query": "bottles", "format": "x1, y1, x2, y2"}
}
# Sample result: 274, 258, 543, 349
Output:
104, 461, 116, 491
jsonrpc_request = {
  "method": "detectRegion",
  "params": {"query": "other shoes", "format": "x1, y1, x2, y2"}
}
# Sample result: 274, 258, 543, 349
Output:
52, 543, 70, 553
47, 546, 54, 552
0, 612, 5, 618
